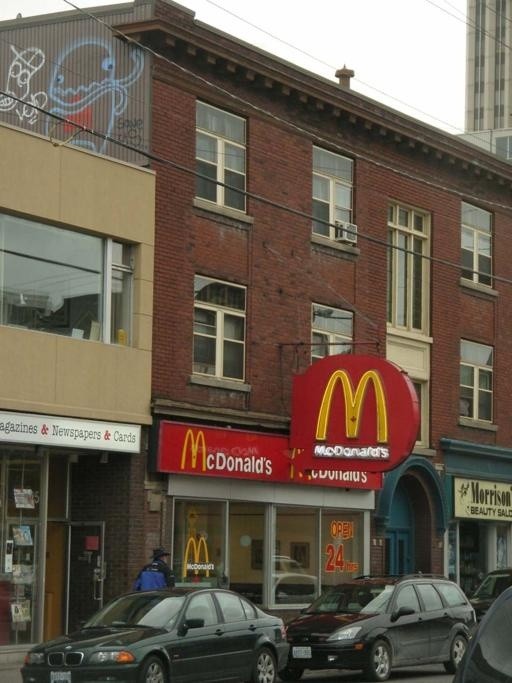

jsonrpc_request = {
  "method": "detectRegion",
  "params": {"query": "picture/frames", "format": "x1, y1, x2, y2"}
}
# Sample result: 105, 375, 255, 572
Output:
250, 538, 280, 570
289, 541, 310, 568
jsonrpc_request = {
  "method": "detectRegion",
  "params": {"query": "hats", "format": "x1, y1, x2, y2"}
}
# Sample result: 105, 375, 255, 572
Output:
150, 548, 170, 558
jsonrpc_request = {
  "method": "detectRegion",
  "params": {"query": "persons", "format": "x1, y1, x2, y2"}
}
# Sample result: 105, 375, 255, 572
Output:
133, 547, 175, 592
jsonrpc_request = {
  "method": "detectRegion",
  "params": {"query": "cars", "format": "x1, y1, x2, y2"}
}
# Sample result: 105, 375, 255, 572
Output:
452, 585, 512, 682
467, 568, 512, 624
20, 587, 290, 682
273, 555, 318, 603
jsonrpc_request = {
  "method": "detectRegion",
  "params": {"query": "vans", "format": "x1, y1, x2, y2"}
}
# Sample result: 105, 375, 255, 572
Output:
278, 572, 478, 683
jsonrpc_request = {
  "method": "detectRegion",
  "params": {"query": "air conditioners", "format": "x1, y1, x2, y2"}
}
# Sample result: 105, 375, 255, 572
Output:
335, 220, 357, 244
